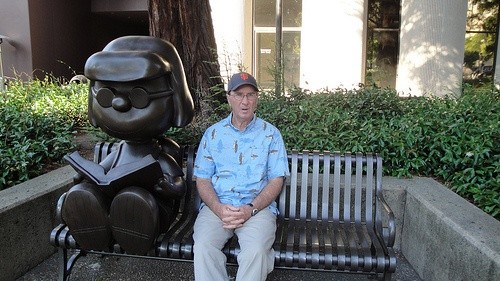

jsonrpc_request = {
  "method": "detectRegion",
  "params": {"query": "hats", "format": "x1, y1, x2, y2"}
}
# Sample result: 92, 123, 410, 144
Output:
228, 72, 260, 94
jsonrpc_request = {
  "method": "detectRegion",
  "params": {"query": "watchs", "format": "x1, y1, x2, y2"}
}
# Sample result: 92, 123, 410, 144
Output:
247, 202, 258, 216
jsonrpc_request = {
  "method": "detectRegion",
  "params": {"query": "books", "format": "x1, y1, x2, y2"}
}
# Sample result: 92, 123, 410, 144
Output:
63, 150, 165, 195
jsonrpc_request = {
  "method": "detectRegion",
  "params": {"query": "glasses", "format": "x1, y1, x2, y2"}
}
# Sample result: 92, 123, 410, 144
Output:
229, 91, 257, 100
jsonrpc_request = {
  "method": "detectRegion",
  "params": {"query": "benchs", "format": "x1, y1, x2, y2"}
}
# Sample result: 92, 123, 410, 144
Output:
50, 140, 397, 281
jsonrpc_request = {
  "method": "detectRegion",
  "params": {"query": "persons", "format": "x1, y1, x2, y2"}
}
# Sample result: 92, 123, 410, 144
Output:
56, 35, 194, 256
192, 72, 291, 281
